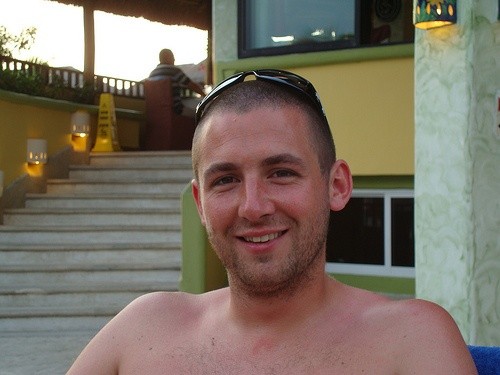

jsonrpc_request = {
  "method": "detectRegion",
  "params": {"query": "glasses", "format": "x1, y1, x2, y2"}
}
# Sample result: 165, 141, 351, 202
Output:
194, 69, 324, 126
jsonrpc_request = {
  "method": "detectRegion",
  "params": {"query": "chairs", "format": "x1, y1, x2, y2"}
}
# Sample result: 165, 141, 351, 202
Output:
143, 79, 197, 150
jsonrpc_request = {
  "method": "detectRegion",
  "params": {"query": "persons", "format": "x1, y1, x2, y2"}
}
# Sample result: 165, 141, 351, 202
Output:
149, 49, 206, 114
65, 67, 477, 375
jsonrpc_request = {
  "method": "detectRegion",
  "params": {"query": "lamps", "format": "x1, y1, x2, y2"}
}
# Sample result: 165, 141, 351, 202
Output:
412, 0, 456, 29
27, 139, 47, 167
70, 112, 90, 141
